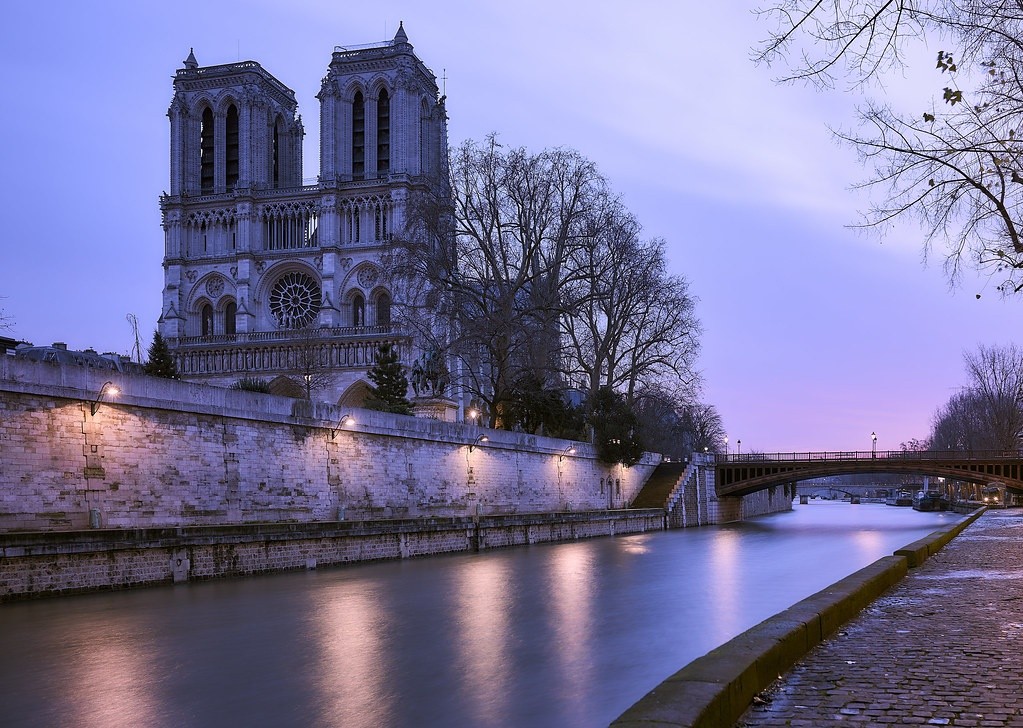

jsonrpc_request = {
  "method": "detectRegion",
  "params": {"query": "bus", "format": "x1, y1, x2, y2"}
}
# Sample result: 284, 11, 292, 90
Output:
900, 492, 911, 498
982, 488, 999, 504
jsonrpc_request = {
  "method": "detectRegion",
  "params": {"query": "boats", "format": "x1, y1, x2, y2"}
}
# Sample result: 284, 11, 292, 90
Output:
912, 490, 942, 512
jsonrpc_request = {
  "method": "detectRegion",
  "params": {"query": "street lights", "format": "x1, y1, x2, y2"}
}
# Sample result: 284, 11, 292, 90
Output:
725, 437, 729, 454
305, 373, 311, 399
874, 438, 877, 451
824, 479, 825, 485
737, 440, 741, 454
470, 411, 476, 426
705, 447, 708, 452
870, 432, 876, 452
